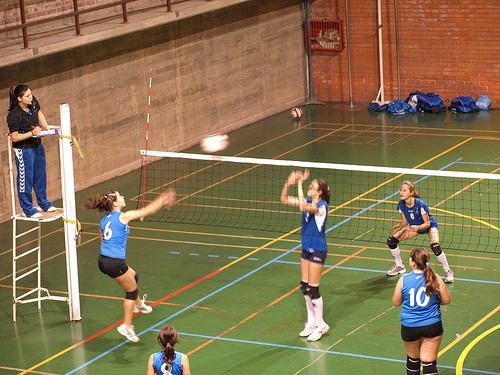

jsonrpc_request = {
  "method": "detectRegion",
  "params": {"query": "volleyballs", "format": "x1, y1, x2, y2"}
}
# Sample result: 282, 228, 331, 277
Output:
198, 133, 230, 153
290, 107, 303, 120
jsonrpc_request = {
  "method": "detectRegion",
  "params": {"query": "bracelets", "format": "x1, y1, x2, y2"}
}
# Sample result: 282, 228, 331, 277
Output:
31, 131, 35, 136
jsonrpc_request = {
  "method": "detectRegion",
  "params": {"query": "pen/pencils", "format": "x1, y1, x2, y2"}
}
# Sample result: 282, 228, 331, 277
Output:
31, 124, 37, 129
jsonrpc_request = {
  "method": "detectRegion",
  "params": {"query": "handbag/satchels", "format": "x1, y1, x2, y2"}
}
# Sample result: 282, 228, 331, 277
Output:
406, 91, 447, 113
450, 96, 479, 113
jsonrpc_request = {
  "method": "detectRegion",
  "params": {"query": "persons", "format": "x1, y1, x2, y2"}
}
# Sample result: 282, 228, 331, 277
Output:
280, 168, 331, 342
7, 84, 57, 218
386, 181, 454, 283
83, 187, 174, 342
147, 325, 191, 375
392, 247, 451, 375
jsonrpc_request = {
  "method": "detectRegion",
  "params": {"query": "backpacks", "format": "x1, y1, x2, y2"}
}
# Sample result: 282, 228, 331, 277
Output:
475, 95, 494, 111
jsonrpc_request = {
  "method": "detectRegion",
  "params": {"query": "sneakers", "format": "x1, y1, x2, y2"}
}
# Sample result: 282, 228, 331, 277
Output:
134, 294, 153, 313
444, 270, 454, 284
117, 323, 140, 343
299, 322, 330, 342
387, 265, 407, 275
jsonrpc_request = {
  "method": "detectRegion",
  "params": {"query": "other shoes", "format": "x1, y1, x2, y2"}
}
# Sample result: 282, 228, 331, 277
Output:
37, 204, 56, 211
21, 211, 43, 217
